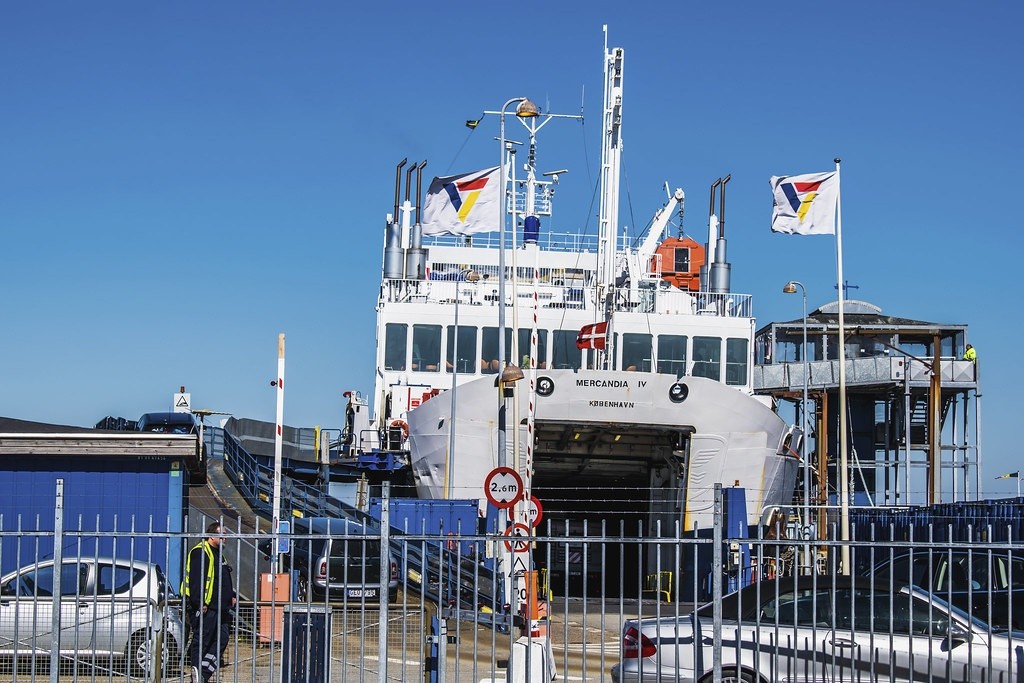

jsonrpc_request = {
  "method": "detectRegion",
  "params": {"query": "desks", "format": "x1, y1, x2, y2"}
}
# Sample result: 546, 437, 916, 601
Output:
460, 360, 469, 372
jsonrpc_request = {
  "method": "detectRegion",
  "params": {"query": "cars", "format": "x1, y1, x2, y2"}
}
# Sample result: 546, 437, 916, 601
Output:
774, 544, 1024, 633
0, 557, 189, 678
137, 413, 207, 484
258, 516, 398, 608
611, 574, 1024, 683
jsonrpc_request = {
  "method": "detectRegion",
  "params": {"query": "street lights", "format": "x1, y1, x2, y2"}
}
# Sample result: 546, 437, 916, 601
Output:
499, 96, 539, 570
784, 281, 810, 597
502, 362, 525, 525
834, 281, 859, 300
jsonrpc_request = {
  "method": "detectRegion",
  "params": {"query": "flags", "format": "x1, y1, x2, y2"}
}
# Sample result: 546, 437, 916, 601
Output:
420, 161, 511, 236
576, 321, 608, 351
768, 171, 837, 234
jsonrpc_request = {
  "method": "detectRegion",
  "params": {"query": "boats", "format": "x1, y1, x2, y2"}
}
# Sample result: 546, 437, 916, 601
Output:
369, 22, 804, 592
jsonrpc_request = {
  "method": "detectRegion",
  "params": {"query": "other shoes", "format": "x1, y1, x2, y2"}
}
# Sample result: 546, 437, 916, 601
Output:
191, 666, 204, 683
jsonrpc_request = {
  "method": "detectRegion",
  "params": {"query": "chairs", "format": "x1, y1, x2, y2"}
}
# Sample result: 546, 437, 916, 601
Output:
446, 361, 454, 372
473, 359, 499, 374
541, 362, 546, 368
627, 366, 636, 371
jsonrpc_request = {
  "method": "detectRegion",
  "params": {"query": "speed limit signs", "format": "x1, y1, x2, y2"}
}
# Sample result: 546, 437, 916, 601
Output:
504, 552, 529, 610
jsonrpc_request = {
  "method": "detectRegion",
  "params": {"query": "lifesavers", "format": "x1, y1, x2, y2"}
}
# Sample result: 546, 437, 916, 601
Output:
390, 420, 409, 443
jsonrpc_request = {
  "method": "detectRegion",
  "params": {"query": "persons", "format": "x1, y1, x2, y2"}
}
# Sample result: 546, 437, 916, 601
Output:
961, 343, 976, 363
180, 521, 236, 683
